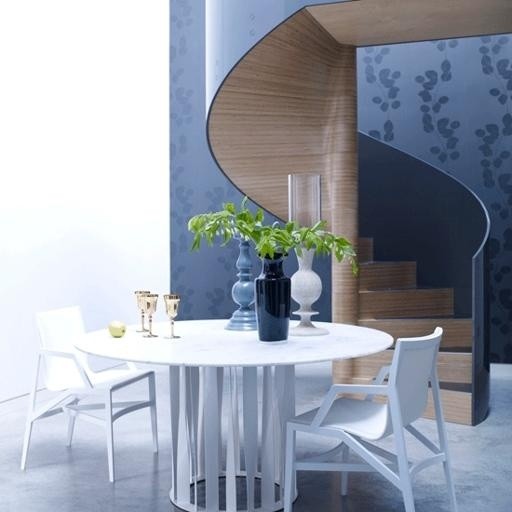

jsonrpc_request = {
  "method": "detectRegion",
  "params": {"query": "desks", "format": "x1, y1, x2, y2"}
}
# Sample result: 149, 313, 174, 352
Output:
77, 319, 394, 512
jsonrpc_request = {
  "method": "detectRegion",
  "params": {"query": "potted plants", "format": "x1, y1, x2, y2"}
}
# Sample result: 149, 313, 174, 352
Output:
187, 196, 358, 342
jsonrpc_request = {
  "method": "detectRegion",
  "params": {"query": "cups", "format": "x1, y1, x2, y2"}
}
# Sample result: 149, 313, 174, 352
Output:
107, 321, 126, 338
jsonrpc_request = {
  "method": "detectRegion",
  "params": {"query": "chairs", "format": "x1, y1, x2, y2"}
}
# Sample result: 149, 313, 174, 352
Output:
21, 306, 159, 481
283, 327, 458, 512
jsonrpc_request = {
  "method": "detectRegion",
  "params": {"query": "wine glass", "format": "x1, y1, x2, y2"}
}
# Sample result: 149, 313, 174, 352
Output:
142, 294, 159, 337
135, 291, 150, 332
164, 295, 181, 338
